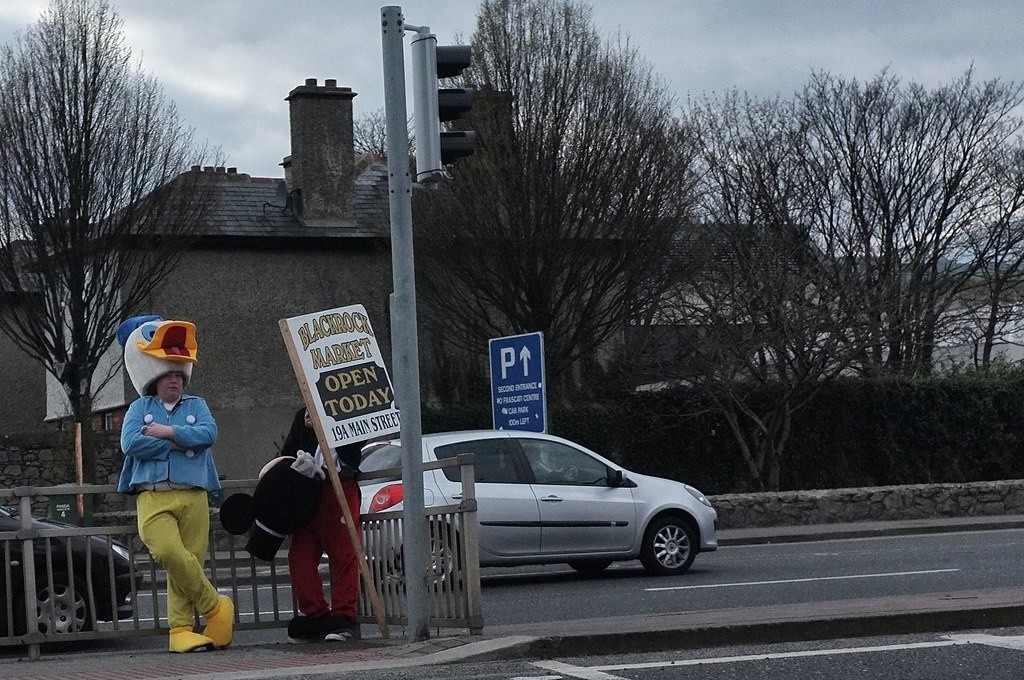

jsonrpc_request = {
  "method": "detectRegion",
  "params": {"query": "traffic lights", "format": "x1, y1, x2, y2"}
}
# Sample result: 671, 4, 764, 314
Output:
411, 33, 477, 185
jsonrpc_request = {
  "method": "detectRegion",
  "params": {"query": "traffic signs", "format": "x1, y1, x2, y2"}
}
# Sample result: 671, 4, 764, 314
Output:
488, 331, 548, 435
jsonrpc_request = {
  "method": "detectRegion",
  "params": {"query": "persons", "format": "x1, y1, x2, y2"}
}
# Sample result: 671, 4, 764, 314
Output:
525, 443, 563, 483
118, 372, 233, 653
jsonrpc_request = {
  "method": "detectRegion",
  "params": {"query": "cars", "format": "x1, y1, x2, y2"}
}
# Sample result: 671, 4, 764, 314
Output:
0, 505, 142, 654
317, 429, 719, 592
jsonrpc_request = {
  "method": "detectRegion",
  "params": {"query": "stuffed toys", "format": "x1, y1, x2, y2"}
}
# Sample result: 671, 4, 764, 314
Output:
245, 405, 365, 642
